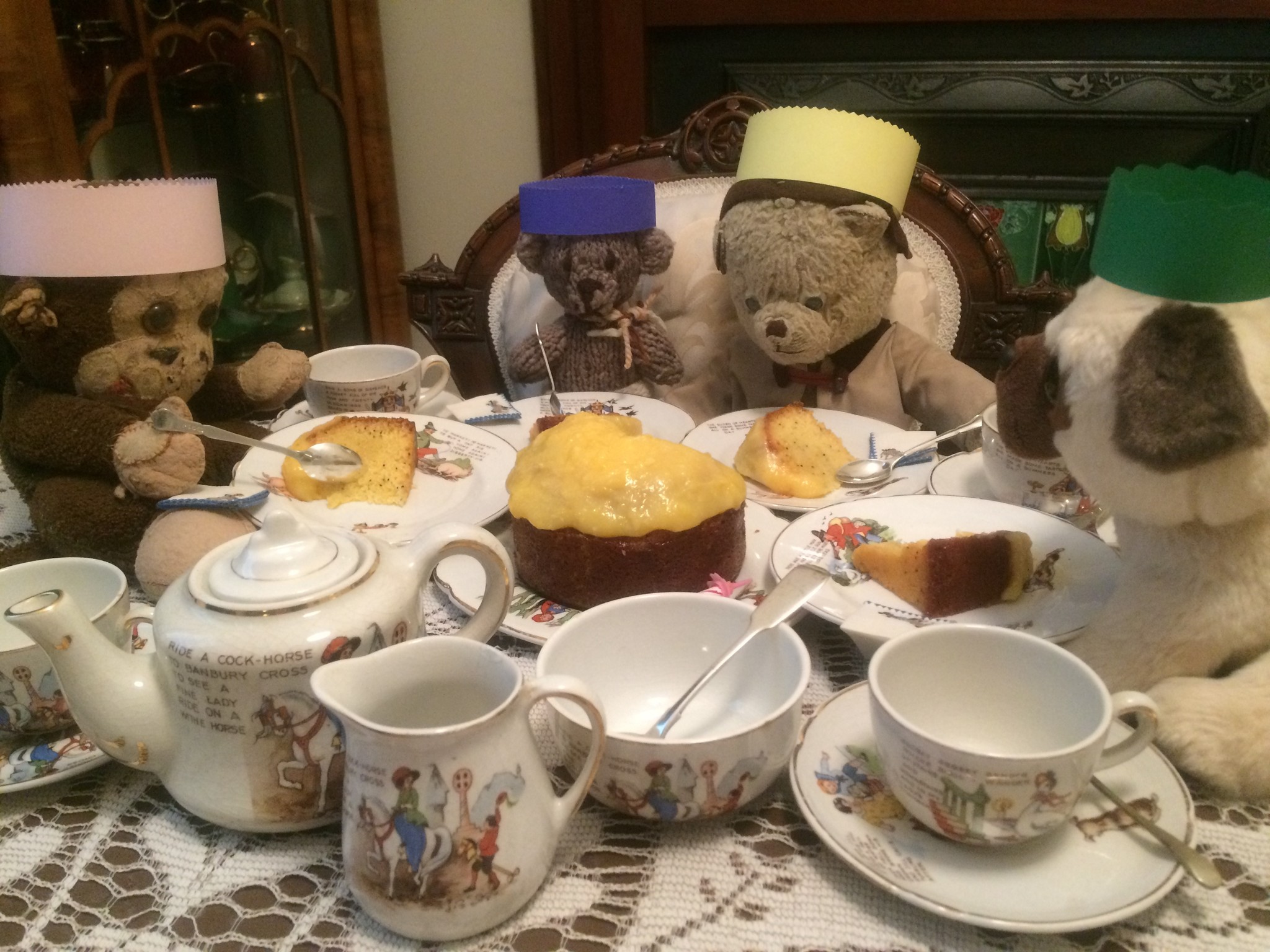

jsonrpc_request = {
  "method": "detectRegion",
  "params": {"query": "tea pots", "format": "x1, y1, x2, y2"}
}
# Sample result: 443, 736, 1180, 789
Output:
5, 510, 516, 835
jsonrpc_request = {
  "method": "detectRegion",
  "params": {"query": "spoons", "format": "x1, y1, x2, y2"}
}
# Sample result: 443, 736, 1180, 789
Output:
1088, 771, 1222, 892
535, 323, 565, 415
149, 407, 364, 484
606, 561, 830, 739
831, 416, 990, 483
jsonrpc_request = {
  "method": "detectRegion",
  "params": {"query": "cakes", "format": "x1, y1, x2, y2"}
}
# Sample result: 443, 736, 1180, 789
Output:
850, 529, 1035, 620
281, 415, 420, 507
735, 400, 860, 498
529, 411, 643, 444
505, 411, 748, 609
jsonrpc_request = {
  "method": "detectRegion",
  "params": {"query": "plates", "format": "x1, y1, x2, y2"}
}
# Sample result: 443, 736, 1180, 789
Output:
680, 405, 1123, 658
0, 602, 153, 795
0, 556, 155, 734
266, 386, 470, 434
473, 388, 695, 451
234, 418, 518, 526
435, 499, 792, 645
789, 678, 1198, 932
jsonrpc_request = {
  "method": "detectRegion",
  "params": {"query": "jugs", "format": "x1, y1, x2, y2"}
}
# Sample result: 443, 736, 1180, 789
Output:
311, 633, 602, 938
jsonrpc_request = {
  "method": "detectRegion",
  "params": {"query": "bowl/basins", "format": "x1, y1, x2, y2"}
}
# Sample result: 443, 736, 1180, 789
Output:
536, 591, 811, 822
981, 402, 1097, 528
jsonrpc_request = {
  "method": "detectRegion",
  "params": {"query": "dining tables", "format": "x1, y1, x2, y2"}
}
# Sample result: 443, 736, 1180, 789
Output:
0, 446, 1270, 951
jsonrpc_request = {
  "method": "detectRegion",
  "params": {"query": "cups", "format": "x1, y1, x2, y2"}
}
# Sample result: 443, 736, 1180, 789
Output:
302, 343, 450, 418
867, 624, 1163, 845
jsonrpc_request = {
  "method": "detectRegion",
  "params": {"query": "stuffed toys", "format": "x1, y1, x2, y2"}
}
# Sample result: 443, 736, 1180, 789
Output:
652, 105, 998, 454
994, 163, 1270, 799
0, 174, 314, 609
505, 174, 686, 394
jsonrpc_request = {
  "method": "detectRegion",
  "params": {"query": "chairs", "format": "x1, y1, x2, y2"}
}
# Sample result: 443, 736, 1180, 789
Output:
398, 94, 1075, 401
